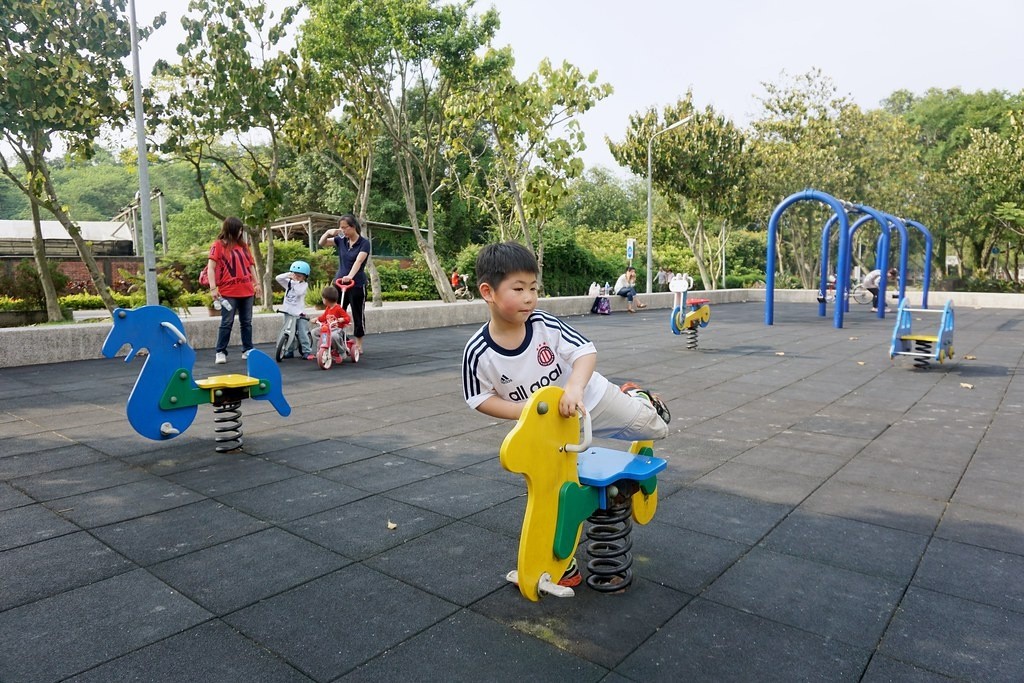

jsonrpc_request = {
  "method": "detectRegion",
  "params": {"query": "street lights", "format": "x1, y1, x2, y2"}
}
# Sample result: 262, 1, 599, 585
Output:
646, 115, 695, 292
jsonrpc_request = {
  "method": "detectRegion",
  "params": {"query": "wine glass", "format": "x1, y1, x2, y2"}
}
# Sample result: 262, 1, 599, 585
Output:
605, 282, 609, 296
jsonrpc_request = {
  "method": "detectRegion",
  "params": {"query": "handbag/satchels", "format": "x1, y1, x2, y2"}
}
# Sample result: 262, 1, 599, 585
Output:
198, 241, 221, 286
589, 282, 601, 296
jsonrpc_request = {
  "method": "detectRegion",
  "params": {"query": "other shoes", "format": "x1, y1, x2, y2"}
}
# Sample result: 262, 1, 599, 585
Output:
627, 309, 636, 313
215, 352, 226, 363
637, 303, 647, 308
242, 349, 253, 359
359, 349, 363, 354
302, 353, 310, 359
283, 351, 294, 358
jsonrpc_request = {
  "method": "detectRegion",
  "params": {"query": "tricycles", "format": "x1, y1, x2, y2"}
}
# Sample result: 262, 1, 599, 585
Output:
313, 277, 360, 370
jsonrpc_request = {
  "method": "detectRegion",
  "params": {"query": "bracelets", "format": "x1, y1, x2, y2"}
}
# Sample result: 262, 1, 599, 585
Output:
210, 287, 217, 290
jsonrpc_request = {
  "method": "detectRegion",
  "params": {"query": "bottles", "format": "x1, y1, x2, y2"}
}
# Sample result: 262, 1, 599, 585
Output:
218, 297, 233, 311
213, 299, 222, 310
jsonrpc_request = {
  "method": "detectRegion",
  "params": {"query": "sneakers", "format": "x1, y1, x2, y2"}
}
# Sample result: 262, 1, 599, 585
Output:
513, 557, 581, 589
621, 382, 670, 424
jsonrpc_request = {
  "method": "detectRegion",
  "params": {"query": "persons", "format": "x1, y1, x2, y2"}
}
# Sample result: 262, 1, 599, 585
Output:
452, 267, 466, 295
318, 215, 370, 355
207, 217, 261, 364
614, 267, 647, 313
653, 266, 675, 292
311, 286, 351, 359
863, 269, 896, 312
275, 260, 314, 360
461, 243, 671, 587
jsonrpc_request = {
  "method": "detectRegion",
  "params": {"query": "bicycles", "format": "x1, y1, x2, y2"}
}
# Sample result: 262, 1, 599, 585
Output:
451, 274, 476, 301
275, 309, 313, 364
824, 273, 874, 305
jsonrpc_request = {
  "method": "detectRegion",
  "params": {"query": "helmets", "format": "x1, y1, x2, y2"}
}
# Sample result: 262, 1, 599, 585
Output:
289, 261, 310, 276
